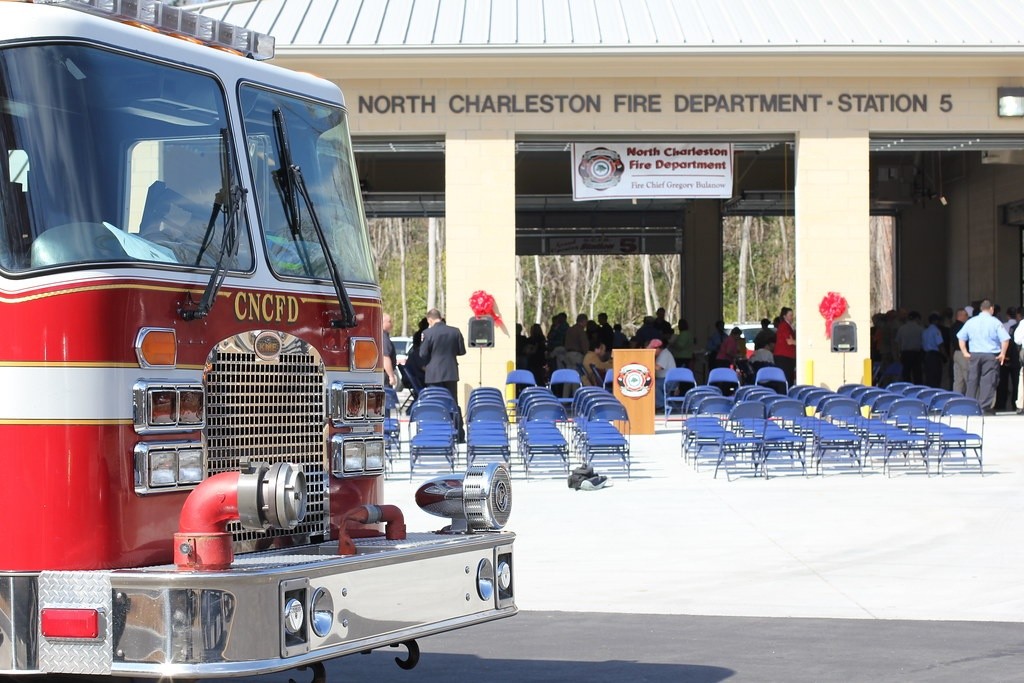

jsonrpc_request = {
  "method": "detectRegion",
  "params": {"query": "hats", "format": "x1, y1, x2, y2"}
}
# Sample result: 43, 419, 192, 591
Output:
392, 365, 404, 392
642, 316, 653, 323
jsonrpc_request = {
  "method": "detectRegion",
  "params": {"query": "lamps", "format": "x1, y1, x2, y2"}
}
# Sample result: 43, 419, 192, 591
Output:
998, 86, 1024, 117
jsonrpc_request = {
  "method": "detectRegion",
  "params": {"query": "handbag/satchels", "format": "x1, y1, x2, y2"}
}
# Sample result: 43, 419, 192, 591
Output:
522, 338, 538, 354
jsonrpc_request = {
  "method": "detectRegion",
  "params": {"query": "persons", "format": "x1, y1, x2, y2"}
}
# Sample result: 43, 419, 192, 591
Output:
630, 307, 693, 411
420, 309, 466, 444
409, 319, 429, 389
517, 312, 630, 395
706, 321, 742, 388
749, 307, 795, 396
870, 299, 1024, 414
382, 313, 396, 416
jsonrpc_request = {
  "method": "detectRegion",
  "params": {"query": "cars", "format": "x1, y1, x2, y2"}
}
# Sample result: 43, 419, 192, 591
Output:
724, 323, 778, 360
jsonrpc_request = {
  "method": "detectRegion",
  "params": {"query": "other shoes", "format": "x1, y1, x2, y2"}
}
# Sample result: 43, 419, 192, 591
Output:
1015, 408, 1023, 414
982, 406, 997, 415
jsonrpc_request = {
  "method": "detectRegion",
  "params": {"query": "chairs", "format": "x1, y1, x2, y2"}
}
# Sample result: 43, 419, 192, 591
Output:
664, 367, 983, 482
385, 369, 631, 479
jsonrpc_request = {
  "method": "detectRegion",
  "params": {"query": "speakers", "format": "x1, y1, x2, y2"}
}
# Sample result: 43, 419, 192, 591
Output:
831, 322, 857, 352
468, 316, 495, 348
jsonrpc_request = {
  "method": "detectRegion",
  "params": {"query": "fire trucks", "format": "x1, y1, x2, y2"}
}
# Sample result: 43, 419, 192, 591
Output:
0, 0, 519, 683
